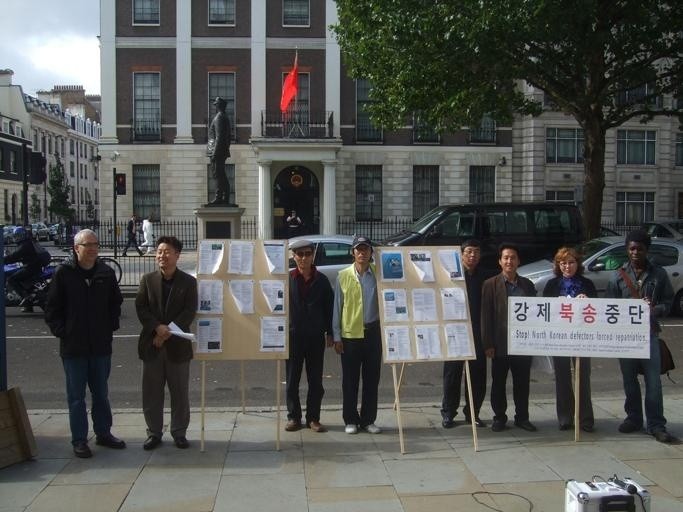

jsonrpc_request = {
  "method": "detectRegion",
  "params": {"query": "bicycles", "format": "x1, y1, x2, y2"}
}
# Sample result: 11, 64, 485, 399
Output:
43, 247, 122, 284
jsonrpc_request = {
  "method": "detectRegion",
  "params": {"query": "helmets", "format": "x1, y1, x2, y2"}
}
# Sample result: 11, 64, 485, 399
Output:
13, 227, 25, 243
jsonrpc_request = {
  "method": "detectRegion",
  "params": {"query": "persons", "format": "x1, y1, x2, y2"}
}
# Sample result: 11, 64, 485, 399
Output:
541, 247, 598, 432
5, 227, 44, 314
332, 234, 383, 437
135, 237, 199, 450
204, 96, 233, 206
597, 252, 619, 271
45, 229, 127, 457
284, 240, 333, 434
605, 232, 680, 444
440, 238, 498, 427
286, 208, 303, 237
481, 246, 537, 432
56, 215, 157, 256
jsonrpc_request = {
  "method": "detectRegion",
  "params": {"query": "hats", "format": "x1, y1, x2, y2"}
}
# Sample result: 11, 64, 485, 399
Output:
353, 237, 372, 248
291, 240, 313, 252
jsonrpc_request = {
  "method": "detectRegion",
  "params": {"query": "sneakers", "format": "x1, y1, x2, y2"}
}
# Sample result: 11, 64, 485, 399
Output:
345, 424, 357, 433
308, 419, 323, 431
364, 423, 381, 433
96, 435, 125, 448
175, 436, 188, 448
144, 436, 157, 449
442, 413, 672, 442
286, 419, 300, 431
73, 444, 91, 457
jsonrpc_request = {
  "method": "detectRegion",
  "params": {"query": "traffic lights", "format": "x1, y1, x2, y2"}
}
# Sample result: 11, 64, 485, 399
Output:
114, 173, 126, 196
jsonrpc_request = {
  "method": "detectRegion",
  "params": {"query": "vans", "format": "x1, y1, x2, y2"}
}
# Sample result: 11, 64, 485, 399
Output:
383, 201, 583, 269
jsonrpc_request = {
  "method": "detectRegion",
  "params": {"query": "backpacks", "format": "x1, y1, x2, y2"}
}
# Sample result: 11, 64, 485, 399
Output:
32, 241, 51, 265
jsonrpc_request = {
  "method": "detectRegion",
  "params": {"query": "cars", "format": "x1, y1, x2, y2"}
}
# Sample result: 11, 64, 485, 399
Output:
640, 221, 682, 238
2, 226, 21, 244
53, 226, 81, 245
515, 236, 683, 314
28, 224, 49, 242
183, 234, 383, 288
47, 223, 59, 240
599, 226, 620, 237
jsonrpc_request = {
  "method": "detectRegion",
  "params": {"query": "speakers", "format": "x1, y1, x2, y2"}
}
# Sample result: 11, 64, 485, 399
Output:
565, 479, 651, 512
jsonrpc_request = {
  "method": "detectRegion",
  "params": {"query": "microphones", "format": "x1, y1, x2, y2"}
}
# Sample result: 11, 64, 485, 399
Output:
609, 477, 637, 494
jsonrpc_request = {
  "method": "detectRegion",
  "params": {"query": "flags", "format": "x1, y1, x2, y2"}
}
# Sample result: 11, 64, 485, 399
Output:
276, 56, 300, 114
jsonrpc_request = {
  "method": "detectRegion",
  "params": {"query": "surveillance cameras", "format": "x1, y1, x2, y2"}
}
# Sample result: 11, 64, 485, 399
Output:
114, 150, 120, 156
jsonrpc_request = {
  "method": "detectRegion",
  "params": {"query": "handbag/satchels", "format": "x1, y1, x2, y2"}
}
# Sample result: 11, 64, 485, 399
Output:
660, 339, 675, 374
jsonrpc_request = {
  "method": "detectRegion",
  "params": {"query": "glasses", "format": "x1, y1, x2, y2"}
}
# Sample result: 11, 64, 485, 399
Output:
297, 252, 311, 256
78, 243, 97, 247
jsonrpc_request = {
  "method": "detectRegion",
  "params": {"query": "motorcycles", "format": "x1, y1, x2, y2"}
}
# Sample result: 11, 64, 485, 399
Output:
2, 249, 56, 313
